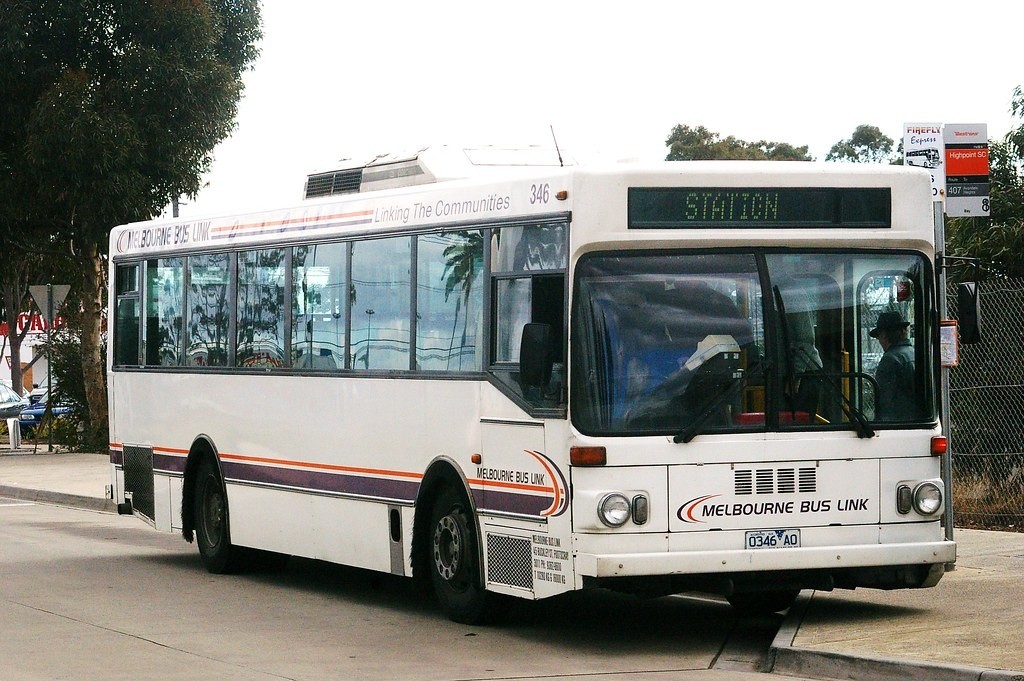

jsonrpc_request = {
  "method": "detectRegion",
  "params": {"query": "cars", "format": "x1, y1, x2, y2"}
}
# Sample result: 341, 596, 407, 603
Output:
18, 384, 86, 430
29, 370, 68, 405
0, 378, 30, 418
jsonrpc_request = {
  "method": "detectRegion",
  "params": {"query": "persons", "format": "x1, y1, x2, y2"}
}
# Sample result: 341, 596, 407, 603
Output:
869, 311, 915, 421
668, 271, 823, 424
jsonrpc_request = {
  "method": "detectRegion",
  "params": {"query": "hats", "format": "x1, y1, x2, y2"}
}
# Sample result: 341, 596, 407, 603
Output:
869, 311, 910, 338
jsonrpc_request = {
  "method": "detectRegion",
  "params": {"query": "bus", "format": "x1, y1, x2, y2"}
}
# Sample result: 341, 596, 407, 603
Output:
905, 147, 942, 169
100, 140, 984, 623
